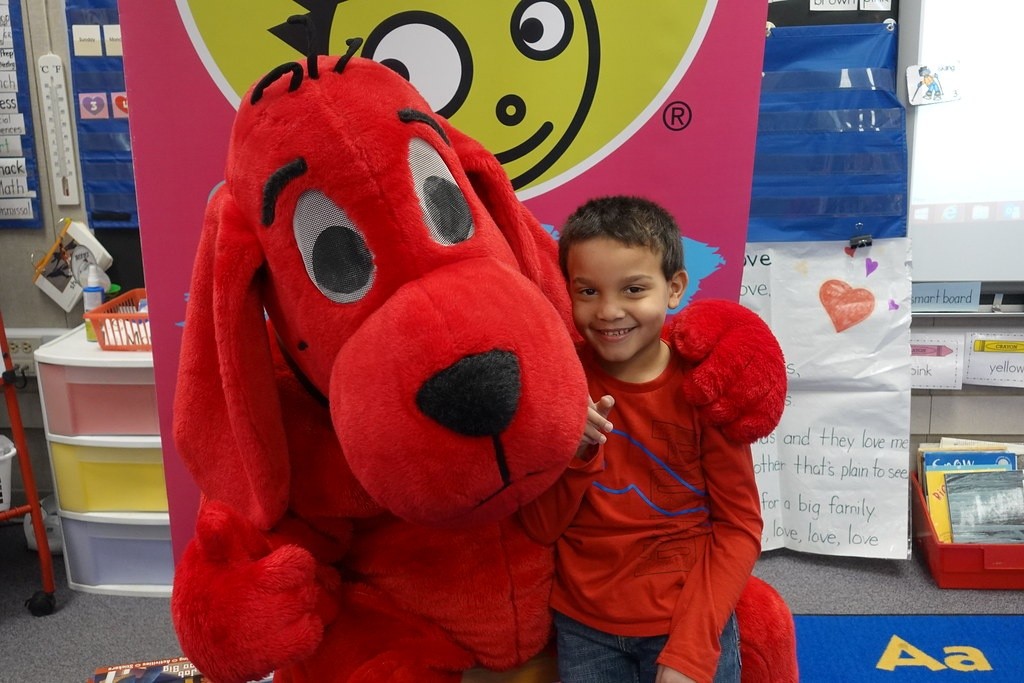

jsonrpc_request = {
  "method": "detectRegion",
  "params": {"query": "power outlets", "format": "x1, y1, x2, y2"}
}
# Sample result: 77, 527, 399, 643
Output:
5, 328, 42, 377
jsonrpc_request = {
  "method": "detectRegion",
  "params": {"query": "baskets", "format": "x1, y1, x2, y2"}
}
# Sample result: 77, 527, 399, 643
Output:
82, 289, 151, 350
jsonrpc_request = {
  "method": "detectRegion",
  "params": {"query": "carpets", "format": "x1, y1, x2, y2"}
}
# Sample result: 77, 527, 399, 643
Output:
787, 612, 1024, 682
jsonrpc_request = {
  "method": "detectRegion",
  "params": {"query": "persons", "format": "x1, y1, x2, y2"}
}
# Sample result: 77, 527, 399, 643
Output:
518, 196, 764, 683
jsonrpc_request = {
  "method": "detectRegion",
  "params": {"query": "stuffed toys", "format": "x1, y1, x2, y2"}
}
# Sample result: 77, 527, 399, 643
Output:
170, 15, 799, 683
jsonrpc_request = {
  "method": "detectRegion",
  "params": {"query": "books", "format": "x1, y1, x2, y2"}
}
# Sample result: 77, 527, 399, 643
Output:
918, 437, 1024, 545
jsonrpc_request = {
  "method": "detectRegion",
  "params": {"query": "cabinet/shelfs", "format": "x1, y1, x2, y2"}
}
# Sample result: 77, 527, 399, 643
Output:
32, 305, 176, 598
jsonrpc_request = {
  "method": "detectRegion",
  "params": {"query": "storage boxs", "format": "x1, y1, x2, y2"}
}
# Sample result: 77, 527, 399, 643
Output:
910, 468, 1024, 591
